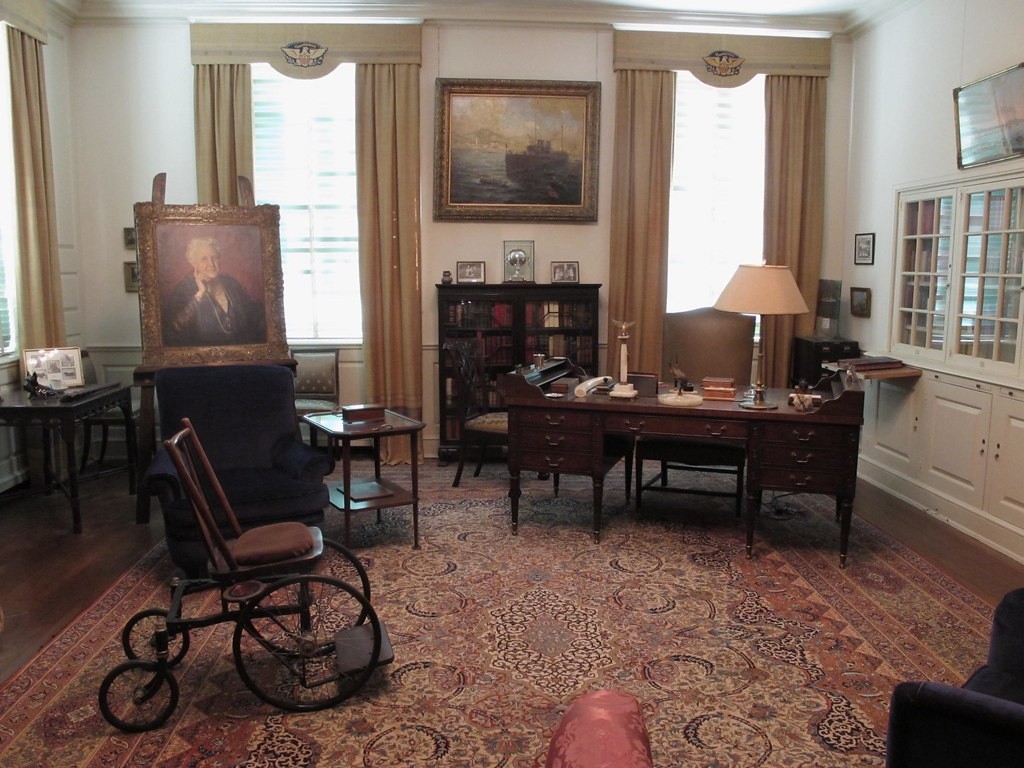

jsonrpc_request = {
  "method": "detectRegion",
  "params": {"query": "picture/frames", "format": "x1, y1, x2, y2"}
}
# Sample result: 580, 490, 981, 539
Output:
18, 347, 83, 390
124, 228, 136, 248
124, 262, 141, 292
954, 63, 1024, 171
851, 287, 872, 317
141, 204, 284, 366
854, 232, 877, 263
551, 261, 579, 283
504, 239, 535, 282
456, 261, 487, 283
432, 79, 599, 223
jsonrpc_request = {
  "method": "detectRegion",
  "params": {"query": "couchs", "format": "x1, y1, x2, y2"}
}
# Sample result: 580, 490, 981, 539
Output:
890, 590, 1024, 768
144, 368, 335, 568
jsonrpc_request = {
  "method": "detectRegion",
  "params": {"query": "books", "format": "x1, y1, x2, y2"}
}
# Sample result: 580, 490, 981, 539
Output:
445, 302, 593, 441
702, 376, 737, 399
838, 356, 902, 371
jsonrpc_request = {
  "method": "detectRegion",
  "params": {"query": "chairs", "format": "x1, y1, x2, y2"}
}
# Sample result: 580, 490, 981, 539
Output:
289, 349, 345, 460
442, 341, 513, 489
79, 349, 143, 495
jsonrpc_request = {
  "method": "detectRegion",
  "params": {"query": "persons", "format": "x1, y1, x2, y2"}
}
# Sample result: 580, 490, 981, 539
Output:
160, 237, 264, 349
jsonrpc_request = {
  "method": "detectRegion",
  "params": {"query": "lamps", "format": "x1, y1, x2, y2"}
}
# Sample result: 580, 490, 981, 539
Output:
714, 263, 810, 398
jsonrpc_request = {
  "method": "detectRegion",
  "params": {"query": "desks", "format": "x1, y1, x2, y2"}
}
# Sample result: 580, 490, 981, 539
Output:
502, 378, 865, 571
0, 386, 138, 534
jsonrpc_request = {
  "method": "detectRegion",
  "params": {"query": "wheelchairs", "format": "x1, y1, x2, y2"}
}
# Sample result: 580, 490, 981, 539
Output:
98, 415, 401, 730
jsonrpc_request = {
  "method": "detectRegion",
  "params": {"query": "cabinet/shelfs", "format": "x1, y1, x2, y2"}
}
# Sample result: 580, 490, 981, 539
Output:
871, 160, 1024, 564
434, 282, 602, 457
795, 335, 860, 388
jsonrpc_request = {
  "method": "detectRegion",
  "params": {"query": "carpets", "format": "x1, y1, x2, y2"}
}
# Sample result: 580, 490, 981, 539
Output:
0, 462, 1001, 768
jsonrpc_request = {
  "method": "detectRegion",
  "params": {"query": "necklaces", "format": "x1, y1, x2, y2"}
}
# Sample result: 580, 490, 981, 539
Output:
205, 284, 234, 335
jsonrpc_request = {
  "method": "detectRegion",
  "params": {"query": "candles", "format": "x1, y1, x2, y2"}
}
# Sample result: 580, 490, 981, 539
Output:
621, 338, 629, 379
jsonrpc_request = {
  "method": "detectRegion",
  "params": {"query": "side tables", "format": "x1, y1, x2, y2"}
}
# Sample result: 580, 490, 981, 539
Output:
300, 410, 427, 549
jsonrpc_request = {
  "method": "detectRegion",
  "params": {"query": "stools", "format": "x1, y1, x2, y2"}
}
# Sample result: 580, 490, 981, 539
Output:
630, 435, 748, 517
